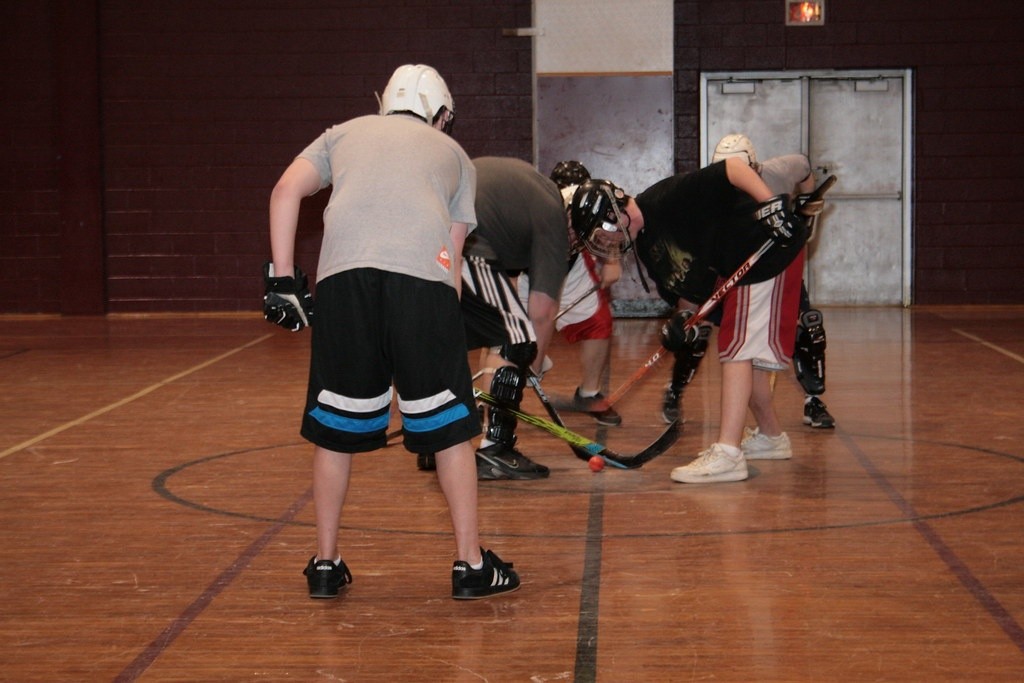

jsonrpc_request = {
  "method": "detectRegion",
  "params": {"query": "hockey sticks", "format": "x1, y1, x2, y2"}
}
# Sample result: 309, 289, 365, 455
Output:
555, 174, 837, 411
263, 293, 682, 471
526, 366, 644, 470
472, 282, 601, 382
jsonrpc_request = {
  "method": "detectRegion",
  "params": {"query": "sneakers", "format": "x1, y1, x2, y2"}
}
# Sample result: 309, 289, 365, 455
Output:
574, 387, 621, 427
670, 442, 748, 484
801, 396, 835, 428
451, 547, 520, 599
474, 434, 550, 481
739, 425, 792, 460
303, 553, 352, 598
663, 383, 683, 424
417, 452, 435, 470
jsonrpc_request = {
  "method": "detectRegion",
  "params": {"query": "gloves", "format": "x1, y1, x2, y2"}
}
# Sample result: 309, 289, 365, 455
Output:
752, 193, 802, 245
793, 194, 823, 242
262, 262, 315, 332
525, 366, 542, 387
658, 310, 701, 353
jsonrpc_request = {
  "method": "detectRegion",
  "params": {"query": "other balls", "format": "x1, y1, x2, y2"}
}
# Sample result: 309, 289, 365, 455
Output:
588, 456, 604, 472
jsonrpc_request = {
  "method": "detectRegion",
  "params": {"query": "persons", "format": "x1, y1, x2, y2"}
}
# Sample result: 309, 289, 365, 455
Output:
264, 65, 521, 600
415, 134, 836, 483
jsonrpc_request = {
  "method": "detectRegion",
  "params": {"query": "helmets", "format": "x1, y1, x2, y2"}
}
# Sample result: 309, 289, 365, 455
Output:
551, 161, 590, 187
378, 63, 454, 120
712, 134, 756, 166
571, 179, 634, 259
